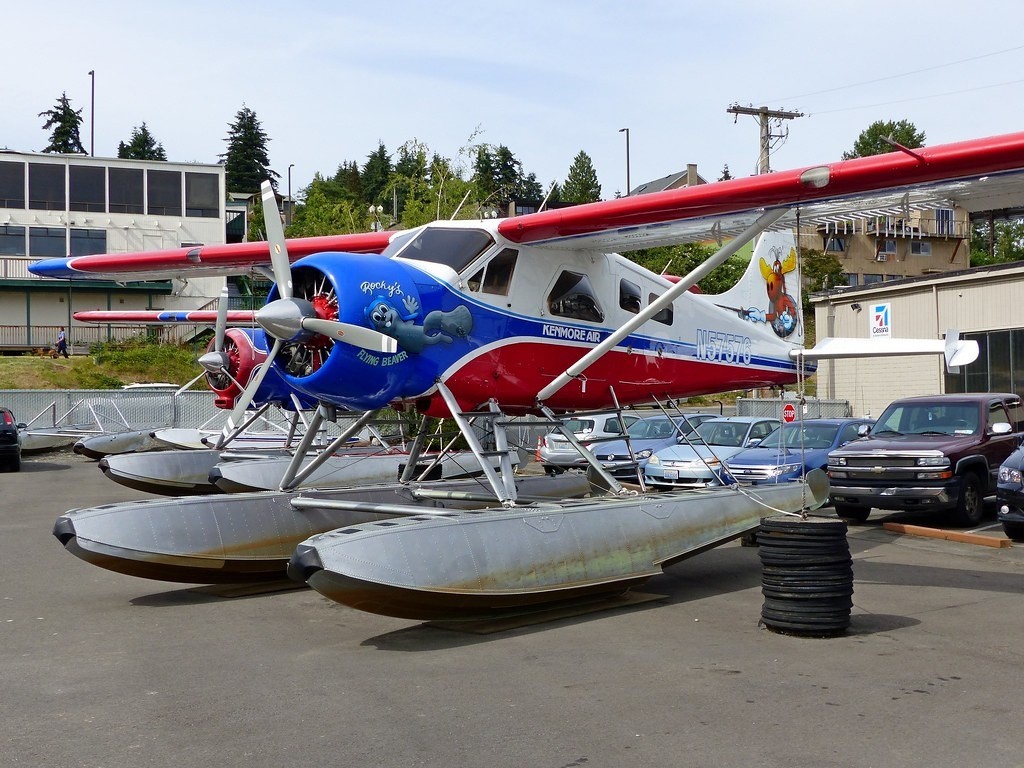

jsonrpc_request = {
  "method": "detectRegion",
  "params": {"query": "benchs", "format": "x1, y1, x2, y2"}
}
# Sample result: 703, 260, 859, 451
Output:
51, 345, 91, 357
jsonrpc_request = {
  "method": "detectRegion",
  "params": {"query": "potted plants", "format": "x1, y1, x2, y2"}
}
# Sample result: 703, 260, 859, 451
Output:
867, 216, 886, 231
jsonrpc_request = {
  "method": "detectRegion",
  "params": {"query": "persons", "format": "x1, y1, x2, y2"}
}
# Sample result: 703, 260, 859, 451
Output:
55, 326, 69, 359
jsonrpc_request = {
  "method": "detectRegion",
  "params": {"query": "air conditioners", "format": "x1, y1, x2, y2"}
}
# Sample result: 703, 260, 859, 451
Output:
877, 255, 887, 262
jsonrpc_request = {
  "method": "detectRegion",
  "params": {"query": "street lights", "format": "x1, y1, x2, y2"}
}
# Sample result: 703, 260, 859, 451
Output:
288, 163, 294, 227
619, 127, 631, 197
87, 70, 95, 157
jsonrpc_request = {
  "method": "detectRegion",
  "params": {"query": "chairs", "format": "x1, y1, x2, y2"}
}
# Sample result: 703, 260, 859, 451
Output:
718, 426, 738, 446
660, 423, 672, 439
749, 426, 762, 439
992, 422, 1012, 434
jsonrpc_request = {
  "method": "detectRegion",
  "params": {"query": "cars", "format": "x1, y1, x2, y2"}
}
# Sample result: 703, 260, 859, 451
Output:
587, 413, 728, 479
996, 444, 1024, 543
0, 407, 27, 473
602, 397, 689, 409
717, 418, 896, 508
540, 415, 664, 477
644, 416, 786, 491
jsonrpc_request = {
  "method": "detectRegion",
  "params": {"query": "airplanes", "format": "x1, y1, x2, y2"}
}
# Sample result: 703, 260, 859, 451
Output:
19, 130, 1024, 620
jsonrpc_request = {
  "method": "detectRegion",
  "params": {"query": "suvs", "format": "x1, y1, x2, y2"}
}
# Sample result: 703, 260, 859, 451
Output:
70, 382, 184, 423
825, 392, 1024, 527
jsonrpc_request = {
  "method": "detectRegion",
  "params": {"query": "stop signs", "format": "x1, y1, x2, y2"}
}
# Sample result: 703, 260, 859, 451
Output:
782, 404, 796, 422
